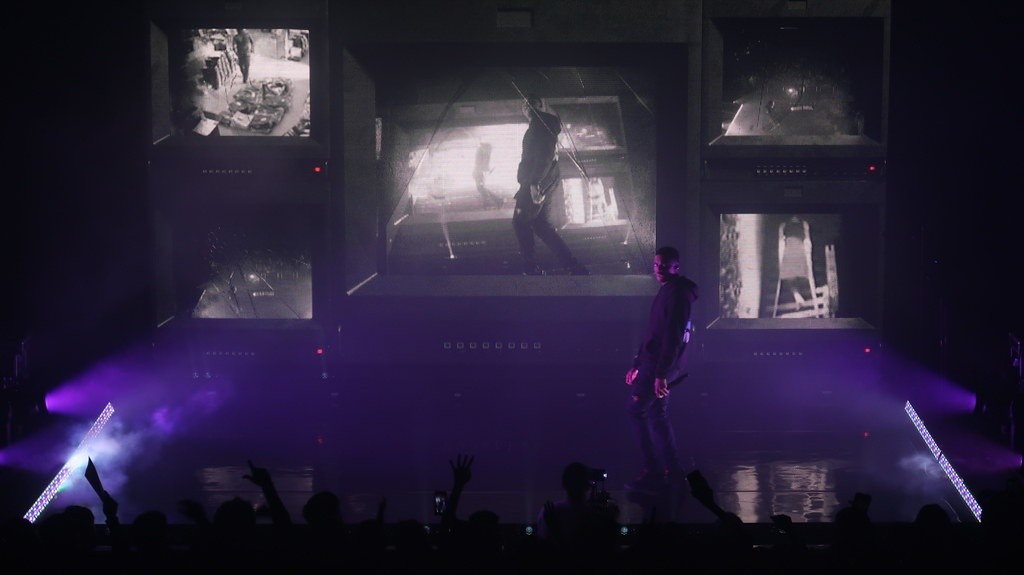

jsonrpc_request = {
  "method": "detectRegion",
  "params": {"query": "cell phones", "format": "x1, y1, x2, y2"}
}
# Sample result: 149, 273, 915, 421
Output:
686, 470, 702, 491
595, 469, 607, 481
854, 493, 867, 505
432, 496, 446, 515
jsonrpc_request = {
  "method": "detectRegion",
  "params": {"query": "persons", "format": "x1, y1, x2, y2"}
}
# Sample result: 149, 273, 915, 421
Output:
0, 454, 1024, 575
625, 247, 698, 485
474, 138, 506, 211
233, 28, 253, 83
511, 94, 590, 278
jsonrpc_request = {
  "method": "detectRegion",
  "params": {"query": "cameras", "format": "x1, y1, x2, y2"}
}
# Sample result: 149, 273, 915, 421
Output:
621, 526, 629, 536
526, 526, 533, 535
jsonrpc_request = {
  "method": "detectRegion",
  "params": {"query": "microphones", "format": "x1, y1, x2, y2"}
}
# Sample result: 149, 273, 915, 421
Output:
667, 373, 689, 390
539, 181, 555, 197
